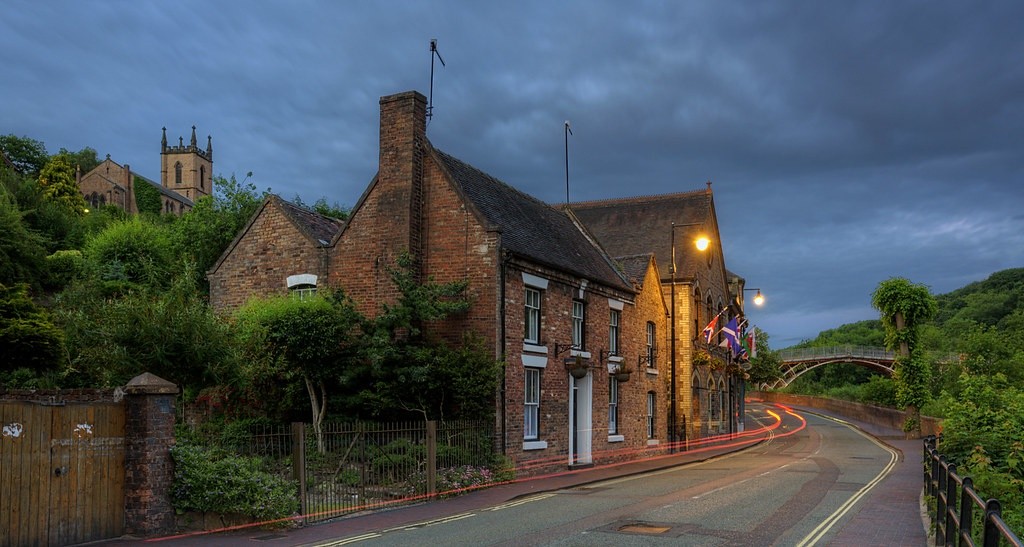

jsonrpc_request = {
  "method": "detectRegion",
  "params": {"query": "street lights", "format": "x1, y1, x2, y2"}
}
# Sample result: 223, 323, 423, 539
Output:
741, 287, 765, 313
669, 220, 710, 454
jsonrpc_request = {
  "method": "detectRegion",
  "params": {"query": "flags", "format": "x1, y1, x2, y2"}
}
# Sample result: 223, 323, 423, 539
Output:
718, 317, 757, 364
702, 316, 718, 344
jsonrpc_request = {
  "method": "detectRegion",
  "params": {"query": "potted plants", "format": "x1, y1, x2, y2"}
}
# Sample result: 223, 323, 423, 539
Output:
569, 354, 587, 379
614, 359, 632, 382
